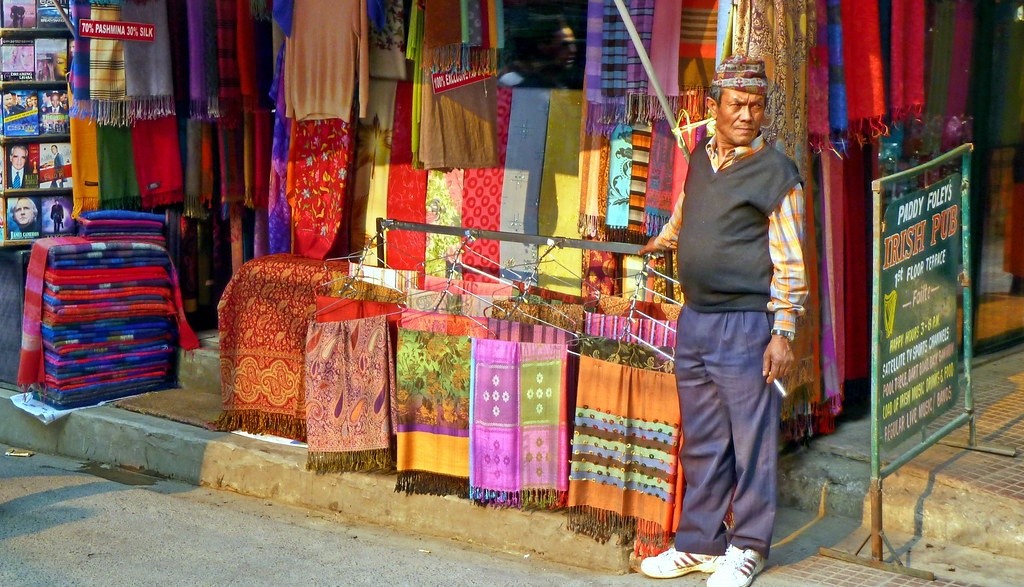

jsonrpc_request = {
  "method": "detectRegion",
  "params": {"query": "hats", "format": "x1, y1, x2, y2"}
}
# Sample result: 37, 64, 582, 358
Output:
711, 57, 768, 95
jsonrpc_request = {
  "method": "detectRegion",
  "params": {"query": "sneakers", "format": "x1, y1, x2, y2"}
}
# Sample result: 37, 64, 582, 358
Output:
706, 544, 764, 587
641, 547, 727, 578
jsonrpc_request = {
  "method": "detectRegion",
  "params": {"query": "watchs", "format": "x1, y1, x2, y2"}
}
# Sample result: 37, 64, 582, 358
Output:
772, 329, 795, 341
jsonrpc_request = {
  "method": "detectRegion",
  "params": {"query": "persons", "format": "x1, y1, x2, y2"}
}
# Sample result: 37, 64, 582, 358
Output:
4, 1, 75, 234
637, 55, 811, 587
497, 12, 583, 90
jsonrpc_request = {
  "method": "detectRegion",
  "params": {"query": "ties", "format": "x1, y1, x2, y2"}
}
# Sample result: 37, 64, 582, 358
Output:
13, 172, 21, 188
54, 109, 56, 113
